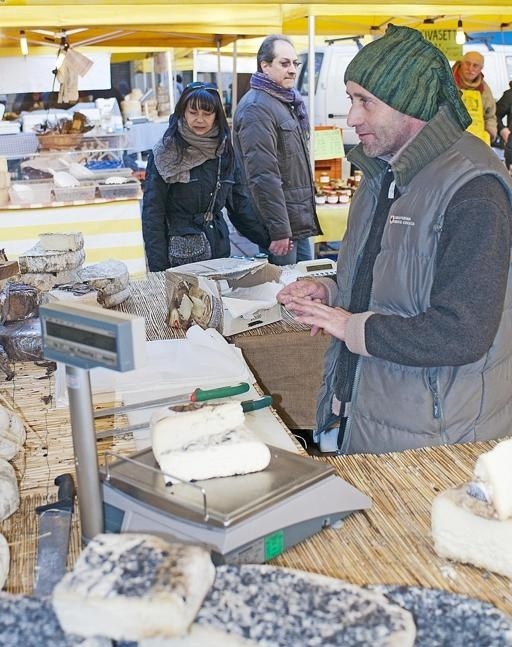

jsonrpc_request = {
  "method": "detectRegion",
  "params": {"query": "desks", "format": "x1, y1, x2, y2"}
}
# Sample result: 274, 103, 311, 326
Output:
313, 190, 350, 252
125, 116, 172, 163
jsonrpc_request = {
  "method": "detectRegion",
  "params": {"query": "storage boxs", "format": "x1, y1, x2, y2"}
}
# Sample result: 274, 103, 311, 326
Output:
162, 257, 284, 337
8, 171, 140, 202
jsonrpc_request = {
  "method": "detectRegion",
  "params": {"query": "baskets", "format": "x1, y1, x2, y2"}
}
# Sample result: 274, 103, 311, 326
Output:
39, 134, 82, 150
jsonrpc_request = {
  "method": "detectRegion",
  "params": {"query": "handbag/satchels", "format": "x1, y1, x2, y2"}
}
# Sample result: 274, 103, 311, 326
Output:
168, 231, 212, 266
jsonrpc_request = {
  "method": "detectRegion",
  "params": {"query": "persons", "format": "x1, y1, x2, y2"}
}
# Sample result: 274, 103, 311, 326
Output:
448, 50, 500, 147
136, 79, 296, 272
228, 35, 324, 266
493, 81, 512, 171
276, 25, 512, 458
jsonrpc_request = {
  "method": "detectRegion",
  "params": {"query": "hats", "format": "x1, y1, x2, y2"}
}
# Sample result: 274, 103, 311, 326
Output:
343, 22, 472, 131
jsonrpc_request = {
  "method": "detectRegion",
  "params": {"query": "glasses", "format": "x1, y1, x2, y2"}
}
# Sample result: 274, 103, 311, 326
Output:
185, 82, 218, 92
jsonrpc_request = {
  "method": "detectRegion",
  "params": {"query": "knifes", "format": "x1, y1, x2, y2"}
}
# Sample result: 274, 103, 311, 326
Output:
32, 470, 74, 598
94, 393, 272, 442
94, 380, 248, 420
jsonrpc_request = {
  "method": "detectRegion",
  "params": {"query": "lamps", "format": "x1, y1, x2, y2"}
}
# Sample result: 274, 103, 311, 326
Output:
456, 15, 466, 44
19, 31, 29, 58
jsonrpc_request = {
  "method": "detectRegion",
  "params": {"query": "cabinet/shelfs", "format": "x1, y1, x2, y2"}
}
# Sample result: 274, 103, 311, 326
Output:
0, 141, 144, 208
308, 125, 342, 191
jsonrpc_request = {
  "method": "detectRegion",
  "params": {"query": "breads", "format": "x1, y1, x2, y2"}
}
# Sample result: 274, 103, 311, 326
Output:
147, 403, 272, 484
1, 230, 133, 362
2, 530, 512, 646
430, 439, 512, 580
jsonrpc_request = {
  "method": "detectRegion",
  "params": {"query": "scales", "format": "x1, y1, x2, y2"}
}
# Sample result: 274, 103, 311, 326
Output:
38, 302, 372, 572
112, 88, 153, 121
